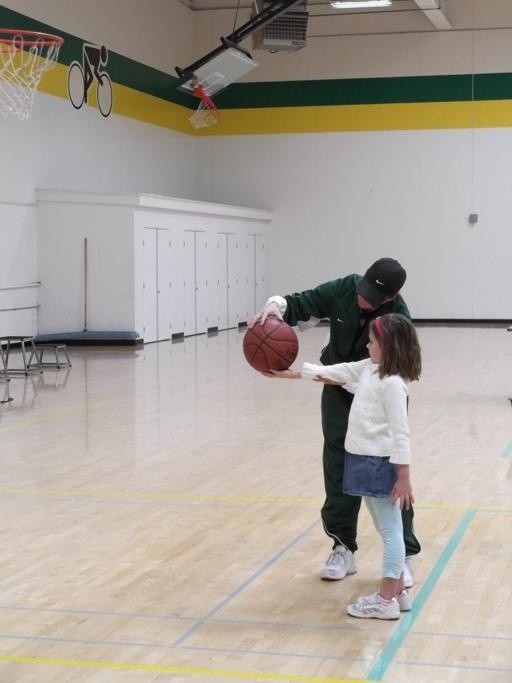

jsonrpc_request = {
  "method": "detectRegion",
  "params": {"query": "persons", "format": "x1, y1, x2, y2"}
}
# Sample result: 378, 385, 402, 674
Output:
248, 256, 422, 581
262, 313, 421, 620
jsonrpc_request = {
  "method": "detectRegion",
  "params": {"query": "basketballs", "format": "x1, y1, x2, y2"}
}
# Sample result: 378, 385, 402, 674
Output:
243, 318, 298, 372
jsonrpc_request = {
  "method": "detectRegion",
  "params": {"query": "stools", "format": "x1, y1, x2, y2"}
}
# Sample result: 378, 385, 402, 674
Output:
27, 342, 73, 370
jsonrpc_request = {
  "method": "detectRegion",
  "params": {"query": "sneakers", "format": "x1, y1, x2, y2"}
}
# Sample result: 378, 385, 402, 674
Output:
321, 544, 357, 580
347, 592, 400, 620
357, 589, 411, 611
403, 557, 414, 587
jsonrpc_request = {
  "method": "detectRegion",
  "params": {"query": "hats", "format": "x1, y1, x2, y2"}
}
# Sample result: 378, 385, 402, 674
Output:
355, 258, 407, 309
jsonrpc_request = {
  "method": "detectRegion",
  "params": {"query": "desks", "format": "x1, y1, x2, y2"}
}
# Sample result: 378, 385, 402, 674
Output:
0, 335, 44, 382
